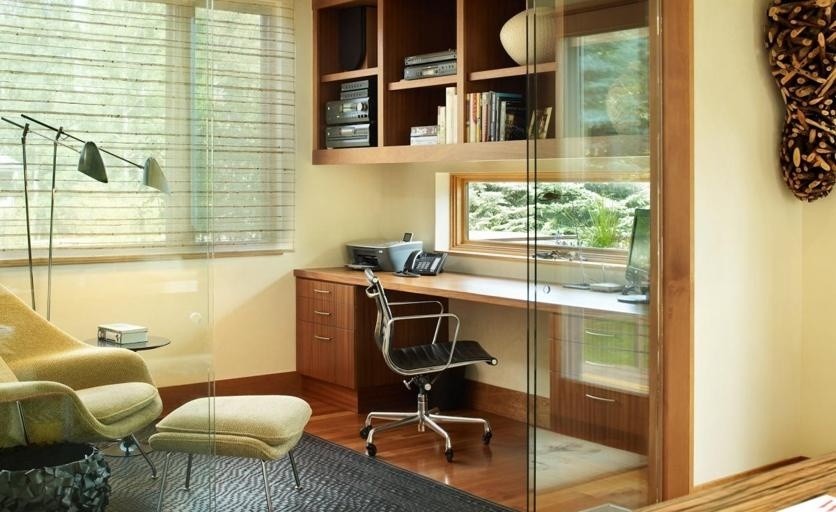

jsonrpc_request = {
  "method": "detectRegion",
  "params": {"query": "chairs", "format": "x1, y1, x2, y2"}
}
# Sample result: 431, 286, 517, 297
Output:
0, 283, 163, 484
360, 267, 499, 462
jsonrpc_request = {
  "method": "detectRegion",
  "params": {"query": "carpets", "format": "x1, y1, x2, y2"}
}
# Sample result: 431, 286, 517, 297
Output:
85, 414, 522, 512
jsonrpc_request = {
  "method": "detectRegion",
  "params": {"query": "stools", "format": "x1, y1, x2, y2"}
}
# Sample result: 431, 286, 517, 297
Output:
148, 394, 312, 512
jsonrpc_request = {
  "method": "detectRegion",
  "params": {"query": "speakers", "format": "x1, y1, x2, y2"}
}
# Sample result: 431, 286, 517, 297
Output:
337, 6, 378, 70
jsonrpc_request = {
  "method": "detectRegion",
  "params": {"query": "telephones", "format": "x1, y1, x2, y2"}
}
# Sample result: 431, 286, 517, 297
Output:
402, 250, 449, 276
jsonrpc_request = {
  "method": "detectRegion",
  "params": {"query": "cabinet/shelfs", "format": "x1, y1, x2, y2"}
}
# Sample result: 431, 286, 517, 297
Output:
312, 0, 652, 182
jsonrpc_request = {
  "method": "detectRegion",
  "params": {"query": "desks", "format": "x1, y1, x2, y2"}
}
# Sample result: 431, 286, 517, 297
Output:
295, 266, 651, 457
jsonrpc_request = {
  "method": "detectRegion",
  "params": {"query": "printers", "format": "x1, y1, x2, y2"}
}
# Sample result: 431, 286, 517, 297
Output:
343, 233, 425, 273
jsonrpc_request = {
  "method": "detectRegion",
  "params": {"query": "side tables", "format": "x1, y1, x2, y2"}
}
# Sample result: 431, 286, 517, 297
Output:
85, 335, 171, 456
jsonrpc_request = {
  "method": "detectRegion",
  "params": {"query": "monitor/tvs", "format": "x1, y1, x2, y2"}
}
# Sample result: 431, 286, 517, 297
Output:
617, 209, 651, 305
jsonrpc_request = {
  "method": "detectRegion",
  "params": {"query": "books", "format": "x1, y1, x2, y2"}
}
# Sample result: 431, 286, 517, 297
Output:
410, 88, 456, 145
465, 91, 524, 144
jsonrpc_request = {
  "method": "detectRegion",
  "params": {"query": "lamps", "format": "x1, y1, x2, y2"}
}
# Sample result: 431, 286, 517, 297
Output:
22, 114, 172, 323
1, 116, 109, 312
